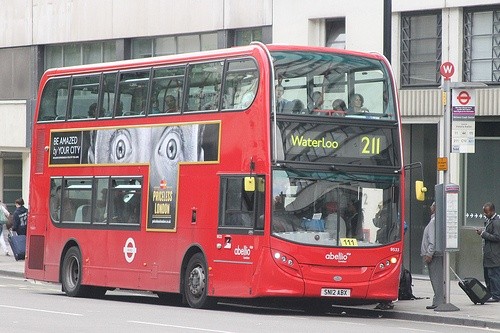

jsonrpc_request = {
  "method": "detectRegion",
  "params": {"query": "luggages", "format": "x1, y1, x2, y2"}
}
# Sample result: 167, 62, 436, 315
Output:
449, 266, 492, 305
8, 228, 26, 261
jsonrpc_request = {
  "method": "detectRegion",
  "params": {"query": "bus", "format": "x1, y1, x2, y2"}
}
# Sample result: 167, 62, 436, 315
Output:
25, 44, 427, 309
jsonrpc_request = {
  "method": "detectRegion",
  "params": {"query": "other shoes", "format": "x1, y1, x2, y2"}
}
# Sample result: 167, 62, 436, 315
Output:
426, 305, 436, 309
374, 302, 394, 310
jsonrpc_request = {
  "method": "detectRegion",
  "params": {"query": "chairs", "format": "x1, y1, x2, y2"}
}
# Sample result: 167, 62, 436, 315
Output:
77, 204, 95, 223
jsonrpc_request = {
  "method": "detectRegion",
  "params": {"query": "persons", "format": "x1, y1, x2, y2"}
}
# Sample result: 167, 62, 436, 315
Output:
374, 204, 408, 244
473, 202, 500, 301
306, 91, 324, 112
332, 93, 369, 112
11, 198, 28, 235
344, 200, 364, 239
150, 96, 162, 114
89, 104, 104, 117
420, 202, 443, 308
273, 85, 285, 113
324, 201, 345, 240
50, 186, 74, 221
0, 200, 10, 255
165, 96, 181, 112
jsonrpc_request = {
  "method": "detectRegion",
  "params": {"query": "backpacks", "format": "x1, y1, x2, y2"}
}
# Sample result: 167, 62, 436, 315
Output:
399, 269, 430, 300
0, 206, 8, 224
